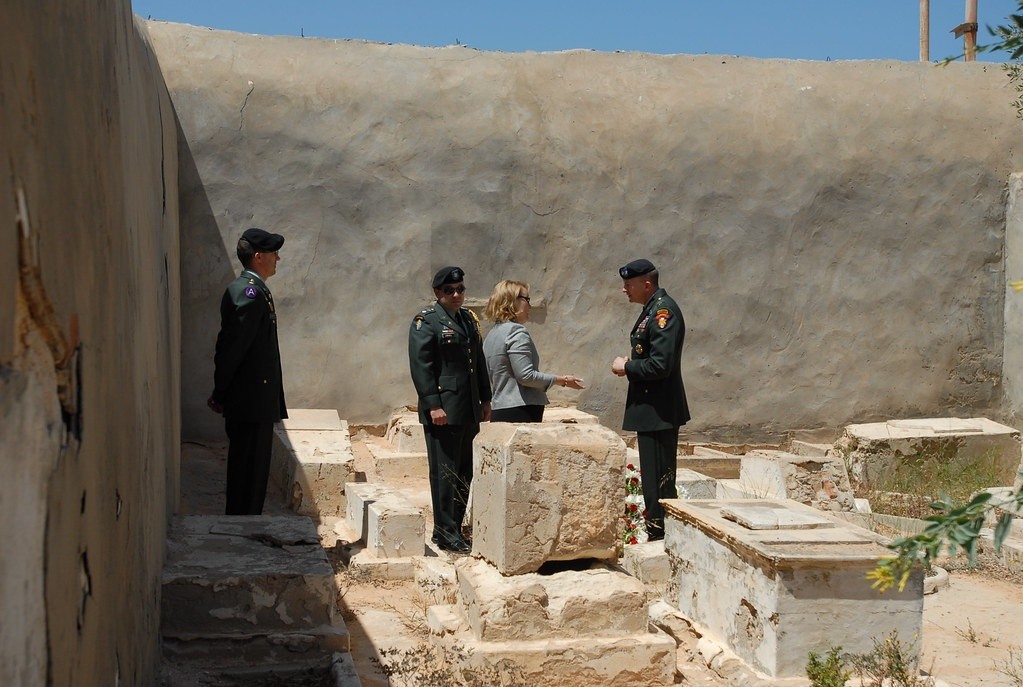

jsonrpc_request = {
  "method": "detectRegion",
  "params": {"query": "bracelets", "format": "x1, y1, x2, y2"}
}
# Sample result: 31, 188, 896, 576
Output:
562, 376, 568, 387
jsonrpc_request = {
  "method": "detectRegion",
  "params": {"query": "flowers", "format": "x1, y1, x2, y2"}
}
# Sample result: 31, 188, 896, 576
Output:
623, 463, 650, 545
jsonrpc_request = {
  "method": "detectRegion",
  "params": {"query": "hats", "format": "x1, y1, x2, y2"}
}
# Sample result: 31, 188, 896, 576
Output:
242, 228, 285, 253
619, 259, 655, 280
432, 267, 465, 288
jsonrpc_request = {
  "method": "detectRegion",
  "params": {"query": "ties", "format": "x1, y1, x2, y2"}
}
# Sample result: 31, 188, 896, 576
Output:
455, 311, 467, 336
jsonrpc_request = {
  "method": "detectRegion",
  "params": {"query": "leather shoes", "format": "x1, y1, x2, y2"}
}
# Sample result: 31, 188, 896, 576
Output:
432, 535, 472, 554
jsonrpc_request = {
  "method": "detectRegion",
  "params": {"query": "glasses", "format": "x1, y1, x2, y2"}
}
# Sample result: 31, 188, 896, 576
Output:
518, 295, 530, 304
436, 284, 466, 295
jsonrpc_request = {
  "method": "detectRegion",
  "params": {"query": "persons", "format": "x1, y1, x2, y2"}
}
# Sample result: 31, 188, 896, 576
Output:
482, 279, 587, 423
208, 228, 289, 516
611, 259, 691, 541
409, 265, 493, 553
